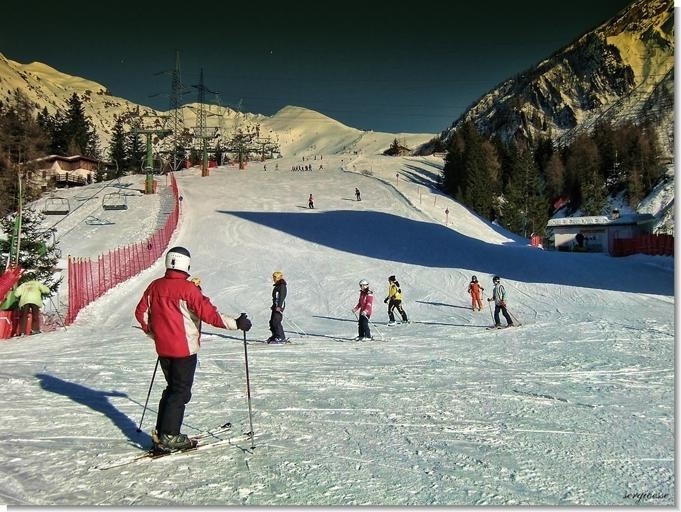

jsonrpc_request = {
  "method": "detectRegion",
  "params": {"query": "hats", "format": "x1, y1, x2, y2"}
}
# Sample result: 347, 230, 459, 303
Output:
493, 276, 500, 280
389, 275, 396, 282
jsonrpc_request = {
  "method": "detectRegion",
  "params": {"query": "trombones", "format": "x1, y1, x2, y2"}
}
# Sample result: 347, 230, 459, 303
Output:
94, 423, 254, 471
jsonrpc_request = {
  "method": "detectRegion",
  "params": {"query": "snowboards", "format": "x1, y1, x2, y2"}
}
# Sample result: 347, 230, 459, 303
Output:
388, 319, 411, 326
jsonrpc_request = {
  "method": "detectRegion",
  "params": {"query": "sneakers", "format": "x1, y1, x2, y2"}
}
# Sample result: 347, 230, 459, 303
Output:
267, 336, 288, 344
355, 336, 374, 341
28, 328, 42, 336
494, 325, 502, 329
387, 320, 397, 326
402, 320, 410, 323
19, 332, 26, 338
505, 323, 514, 328
149, 430, 198, 457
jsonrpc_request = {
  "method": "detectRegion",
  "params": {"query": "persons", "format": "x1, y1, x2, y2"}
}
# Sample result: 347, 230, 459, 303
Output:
263, 154, 324, 172
187, 278, 203, 290
384, 276, 409, 325
14, 280, 51, 336
487, 276, 514, 328
355, 188, 361, 201
309, 194, 314, 209
468, 275, 484, 311
267, 272, 287, 343
135, 247, 252, 453
352, 279, 374, 341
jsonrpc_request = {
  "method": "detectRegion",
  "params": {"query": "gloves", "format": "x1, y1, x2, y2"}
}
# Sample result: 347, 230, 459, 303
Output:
361, 311, 368, 317
384, 297, 389, 303
235, 312, 253, 332
351, 307, 358, 313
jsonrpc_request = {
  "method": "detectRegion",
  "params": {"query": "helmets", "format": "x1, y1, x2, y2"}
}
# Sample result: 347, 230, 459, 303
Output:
191, 277, 201, 287
273, 272, 283, 279
164, 246, 191, 274
359, 279, 370, 291
27, 272, 37, 279
